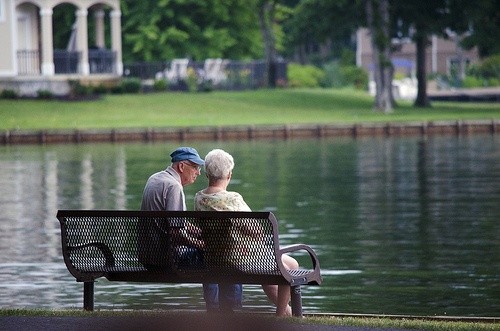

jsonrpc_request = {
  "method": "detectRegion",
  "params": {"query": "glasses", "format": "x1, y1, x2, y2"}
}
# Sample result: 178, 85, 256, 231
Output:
180, 160, 202, 171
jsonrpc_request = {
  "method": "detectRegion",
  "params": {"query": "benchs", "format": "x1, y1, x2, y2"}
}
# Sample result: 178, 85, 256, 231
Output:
56, 210, 323, 319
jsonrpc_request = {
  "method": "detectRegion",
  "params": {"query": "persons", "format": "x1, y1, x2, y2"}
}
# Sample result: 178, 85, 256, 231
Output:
136, 146, 246, 313
192, 149, 300, 317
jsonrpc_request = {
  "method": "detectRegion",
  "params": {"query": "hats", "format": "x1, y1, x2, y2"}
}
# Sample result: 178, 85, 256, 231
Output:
170, 147, 206, 165
